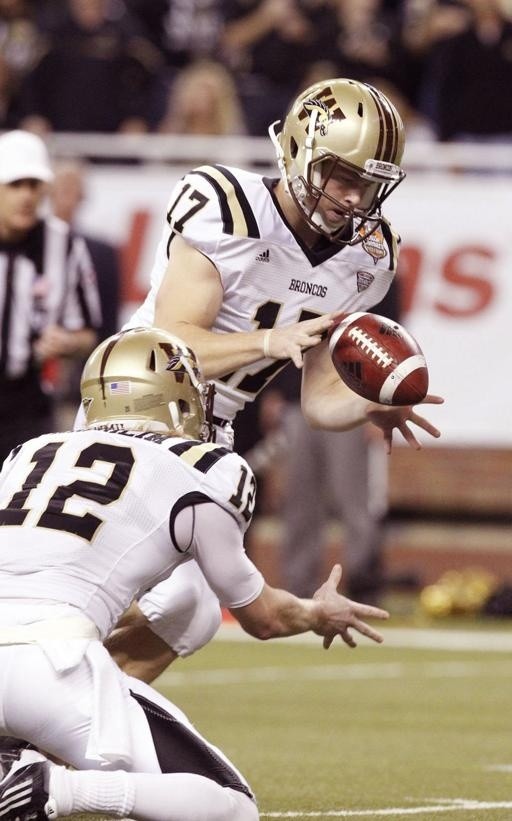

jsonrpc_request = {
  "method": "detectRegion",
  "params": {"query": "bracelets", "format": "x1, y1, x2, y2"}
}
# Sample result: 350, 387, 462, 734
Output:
264, 329, 274, 359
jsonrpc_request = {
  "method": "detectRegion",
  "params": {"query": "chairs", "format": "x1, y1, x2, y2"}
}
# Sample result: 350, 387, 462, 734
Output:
0, 744, 60, 821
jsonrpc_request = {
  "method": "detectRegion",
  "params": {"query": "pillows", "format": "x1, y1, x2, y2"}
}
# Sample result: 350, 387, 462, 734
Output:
280, 77, 407, 246
78, 326, 218, 444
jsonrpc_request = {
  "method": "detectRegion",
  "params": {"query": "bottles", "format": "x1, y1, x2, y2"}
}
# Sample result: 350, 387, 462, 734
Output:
0, 127, 54, 185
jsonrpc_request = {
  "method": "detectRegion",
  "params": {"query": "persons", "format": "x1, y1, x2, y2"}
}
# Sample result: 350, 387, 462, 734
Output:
0, 330, 390, 821
76, 72, 447, 683
0, 129, 102, 470
258, 271, 401, 600
0, 1, 512, 147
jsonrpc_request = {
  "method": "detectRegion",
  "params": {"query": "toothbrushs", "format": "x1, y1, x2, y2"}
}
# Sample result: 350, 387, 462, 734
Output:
327, 311, 429, 407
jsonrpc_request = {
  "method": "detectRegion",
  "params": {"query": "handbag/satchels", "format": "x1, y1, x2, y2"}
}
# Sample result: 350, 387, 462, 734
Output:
264, 329, 274, 359
